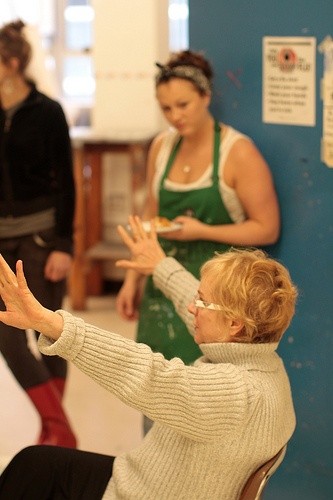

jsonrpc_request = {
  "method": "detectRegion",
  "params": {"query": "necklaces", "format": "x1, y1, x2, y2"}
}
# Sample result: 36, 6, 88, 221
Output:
177, 137, 209, 175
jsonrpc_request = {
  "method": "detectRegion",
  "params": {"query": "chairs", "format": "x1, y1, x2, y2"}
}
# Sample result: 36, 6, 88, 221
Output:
240, 445, 287, 500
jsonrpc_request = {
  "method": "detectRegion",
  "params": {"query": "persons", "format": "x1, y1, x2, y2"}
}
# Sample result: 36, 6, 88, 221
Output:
116, 51, 286, 439
0, 18, 77, 449
0, 212, 298, 499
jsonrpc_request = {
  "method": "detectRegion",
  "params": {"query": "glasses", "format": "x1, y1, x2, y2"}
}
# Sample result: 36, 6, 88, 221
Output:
193, 292, 233, 312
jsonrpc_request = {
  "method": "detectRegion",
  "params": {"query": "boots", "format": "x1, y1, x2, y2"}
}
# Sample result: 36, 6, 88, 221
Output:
26, 378, 77, 448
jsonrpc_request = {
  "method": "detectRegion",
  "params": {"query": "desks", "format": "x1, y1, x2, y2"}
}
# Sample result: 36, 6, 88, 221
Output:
71, 127, 158, 313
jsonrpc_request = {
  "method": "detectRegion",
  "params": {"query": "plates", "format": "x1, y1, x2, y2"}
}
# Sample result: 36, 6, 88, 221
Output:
126, 222, 182, 232
